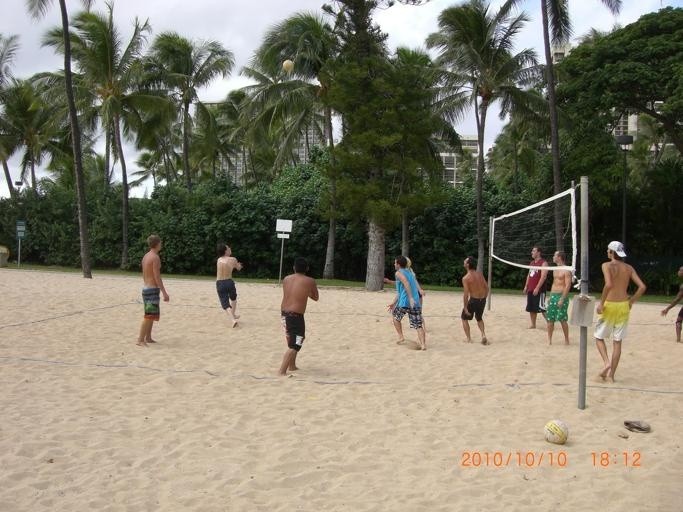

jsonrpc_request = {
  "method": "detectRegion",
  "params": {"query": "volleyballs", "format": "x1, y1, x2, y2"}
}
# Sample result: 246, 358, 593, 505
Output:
544, 419, 567, 444
283, 60, 293, 71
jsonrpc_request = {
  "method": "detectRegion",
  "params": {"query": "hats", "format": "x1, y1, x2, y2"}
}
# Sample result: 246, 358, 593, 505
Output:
608, 240, 627, 258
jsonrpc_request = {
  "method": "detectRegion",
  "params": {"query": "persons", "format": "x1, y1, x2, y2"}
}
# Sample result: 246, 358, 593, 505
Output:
522, 247, 549, 329
383, 255, 427, 351
544, 251, 572, 346
660, 266, 683, 343
460, 256, 489, 345
593, 240, 647, 383
215, 243, 243, 329
136, 234, 169, 348
276, 257, 319, 379
